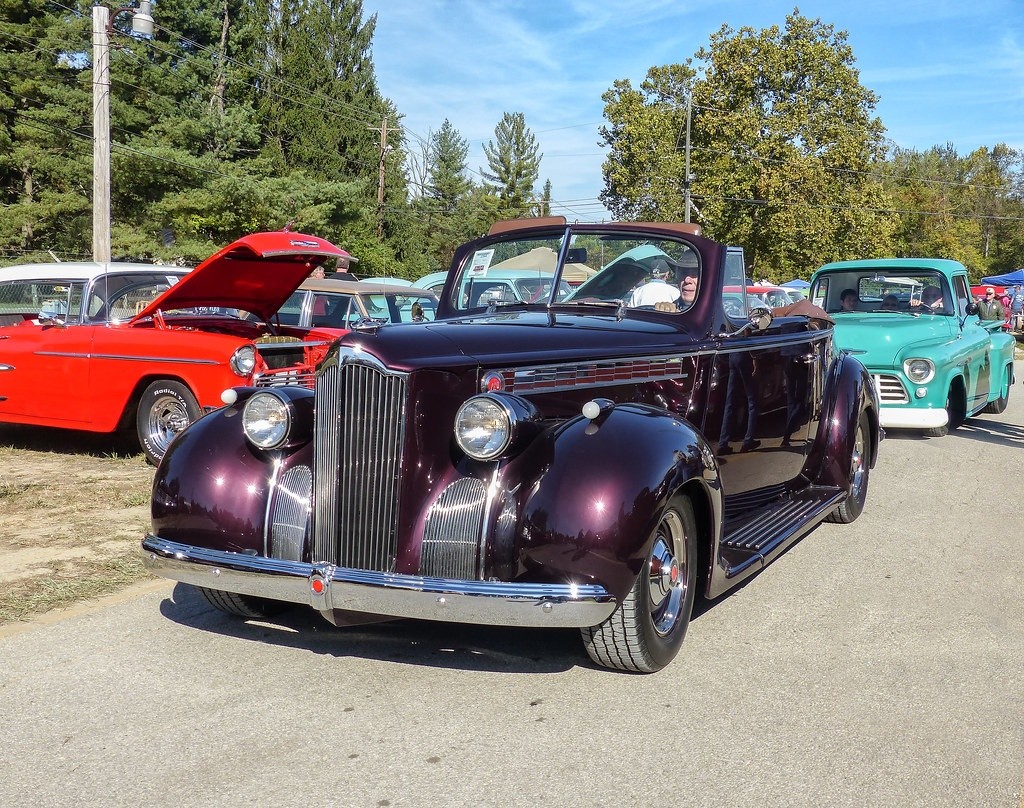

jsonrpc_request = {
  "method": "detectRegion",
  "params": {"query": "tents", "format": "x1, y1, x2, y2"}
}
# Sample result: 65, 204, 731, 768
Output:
865, 277, 923, 300
753, 279, 778, 287
489, 246, 598, 285
780, 279, 811, 288
981, 269, 1024, 286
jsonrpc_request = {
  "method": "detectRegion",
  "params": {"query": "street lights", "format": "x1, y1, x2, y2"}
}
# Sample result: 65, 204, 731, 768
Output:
92, 2, 154, 317
378, 143, 393, 224
684, 173, 696, 224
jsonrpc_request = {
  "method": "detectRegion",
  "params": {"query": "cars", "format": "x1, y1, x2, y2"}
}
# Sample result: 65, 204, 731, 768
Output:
0, 215, 1017, 674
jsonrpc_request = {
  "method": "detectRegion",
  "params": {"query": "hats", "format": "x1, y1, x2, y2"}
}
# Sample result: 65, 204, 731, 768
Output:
985, 288, 995, 294
650, 258, 669, 273
665, 249, 699, 274
1013, 284, 1021, 288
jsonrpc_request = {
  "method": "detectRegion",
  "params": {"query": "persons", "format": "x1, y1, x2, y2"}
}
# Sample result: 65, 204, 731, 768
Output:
299, 258, 359, 315
881, 295, 899, 310
1009, 286, 1024, 327
911, 287, 920, 300
627, 260, 680, 308
898, 289, 906, 297
830, 289, 858, 312
655, 249, 731, 312
999, 289, 1011, 307
976, 287, 1004, 320
877, 288, 890, 299
908, 285, 943, 310
994, 294, 1001, 303
972, 295, 979, 303
716, 323, 811, 454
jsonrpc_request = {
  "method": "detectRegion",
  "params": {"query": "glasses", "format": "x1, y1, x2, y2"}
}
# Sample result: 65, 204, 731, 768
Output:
881, 302, 898, 308
923, 293, 937, 300
986, 293, 993, 295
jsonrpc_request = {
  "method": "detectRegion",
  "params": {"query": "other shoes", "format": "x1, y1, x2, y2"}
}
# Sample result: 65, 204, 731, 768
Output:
740, 440, 761, 452
779, 442, 791, 448
716, 447, 733, 455
